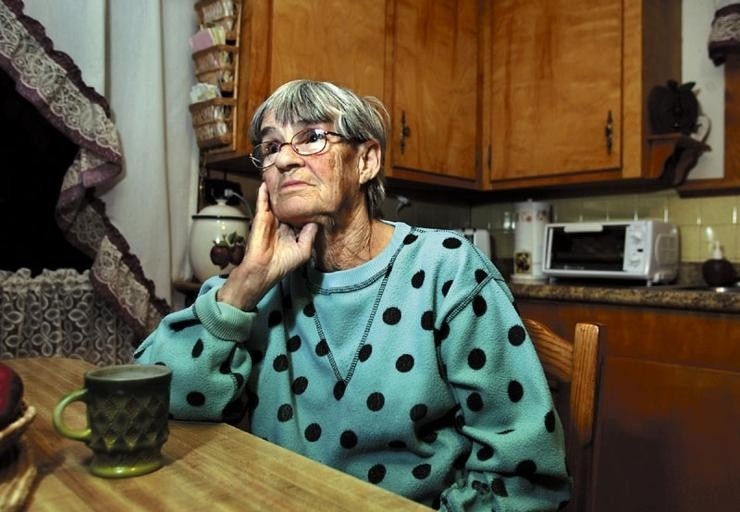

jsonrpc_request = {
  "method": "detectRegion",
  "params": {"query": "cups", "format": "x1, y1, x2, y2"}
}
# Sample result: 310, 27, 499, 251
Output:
52, 363, 177, 479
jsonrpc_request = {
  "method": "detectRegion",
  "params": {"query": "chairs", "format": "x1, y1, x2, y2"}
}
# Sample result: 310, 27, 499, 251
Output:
523, 315, 605, 511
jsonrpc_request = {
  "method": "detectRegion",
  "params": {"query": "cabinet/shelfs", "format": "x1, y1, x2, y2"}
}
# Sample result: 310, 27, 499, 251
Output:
272, 1, 478, 179
490, 1, 623, 183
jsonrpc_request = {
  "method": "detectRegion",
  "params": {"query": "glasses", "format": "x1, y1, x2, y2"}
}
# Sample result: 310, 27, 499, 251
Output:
248, 127, 345, 169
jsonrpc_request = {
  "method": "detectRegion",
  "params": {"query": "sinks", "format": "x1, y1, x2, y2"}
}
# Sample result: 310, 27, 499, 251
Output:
680, 286, 740, 292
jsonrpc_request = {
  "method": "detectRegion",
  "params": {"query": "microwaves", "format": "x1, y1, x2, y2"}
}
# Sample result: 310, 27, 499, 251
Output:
544, 219, 681, 285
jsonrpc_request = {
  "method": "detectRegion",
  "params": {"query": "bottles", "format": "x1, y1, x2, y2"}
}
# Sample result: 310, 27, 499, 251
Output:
510, 196, 554, 287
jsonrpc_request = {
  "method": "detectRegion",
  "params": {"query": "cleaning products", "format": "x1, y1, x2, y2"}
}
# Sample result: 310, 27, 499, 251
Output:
702, 242, 736, 287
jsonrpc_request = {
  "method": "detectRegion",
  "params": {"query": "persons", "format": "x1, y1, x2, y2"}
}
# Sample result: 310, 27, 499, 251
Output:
128, 79, 575, 512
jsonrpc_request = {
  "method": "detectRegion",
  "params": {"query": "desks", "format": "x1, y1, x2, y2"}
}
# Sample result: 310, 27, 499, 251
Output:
0, 358, 439, 511
